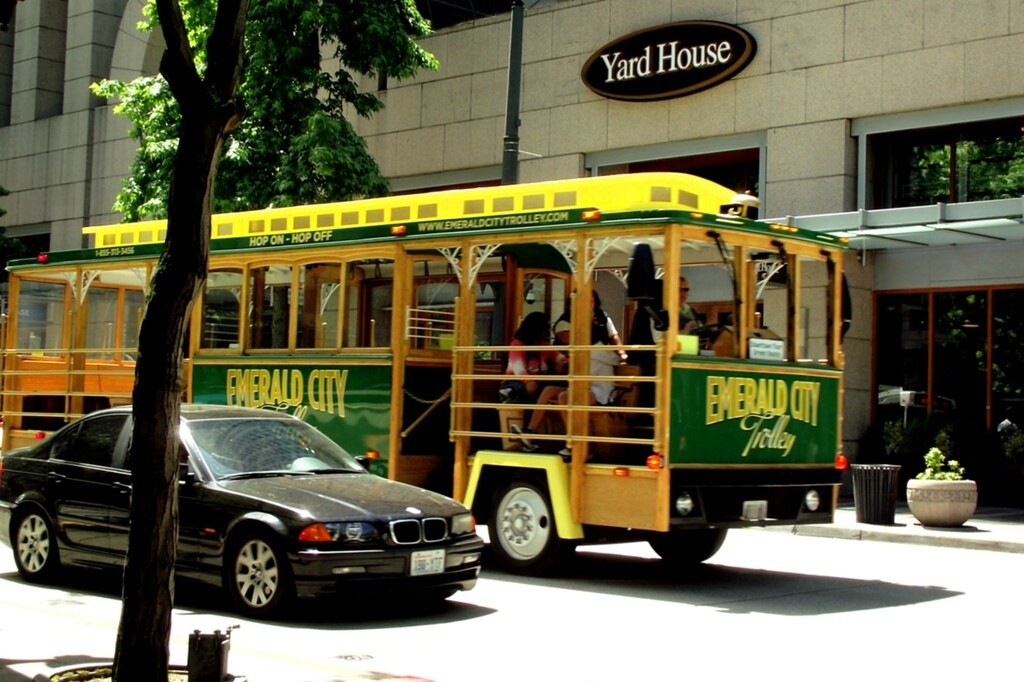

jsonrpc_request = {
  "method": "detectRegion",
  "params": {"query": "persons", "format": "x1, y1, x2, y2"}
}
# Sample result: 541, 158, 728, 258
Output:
502, 244, 664, 458
680, 277, 703, 335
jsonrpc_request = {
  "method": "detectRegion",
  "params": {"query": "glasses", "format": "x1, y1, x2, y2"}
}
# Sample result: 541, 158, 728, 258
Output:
680, 287, 689, 292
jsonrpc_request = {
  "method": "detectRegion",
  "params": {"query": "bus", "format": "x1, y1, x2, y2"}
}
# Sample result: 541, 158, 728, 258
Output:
1, 172, 852, 576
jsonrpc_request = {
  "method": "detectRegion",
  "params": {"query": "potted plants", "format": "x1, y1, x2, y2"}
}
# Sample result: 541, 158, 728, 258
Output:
907, 448, 977, 529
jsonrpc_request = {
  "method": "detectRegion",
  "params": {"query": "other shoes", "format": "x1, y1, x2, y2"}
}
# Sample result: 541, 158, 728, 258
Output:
511, 424, 539, 452
557, 447, 572, 456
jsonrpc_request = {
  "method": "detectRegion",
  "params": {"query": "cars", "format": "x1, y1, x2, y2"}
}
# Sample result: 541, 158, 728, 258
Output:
0, 404, 484, 620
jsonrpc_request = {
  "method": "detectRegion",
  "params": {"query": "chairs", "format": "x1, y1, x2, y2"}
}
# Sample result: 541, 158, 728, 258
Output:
548, 366, 643, 446
